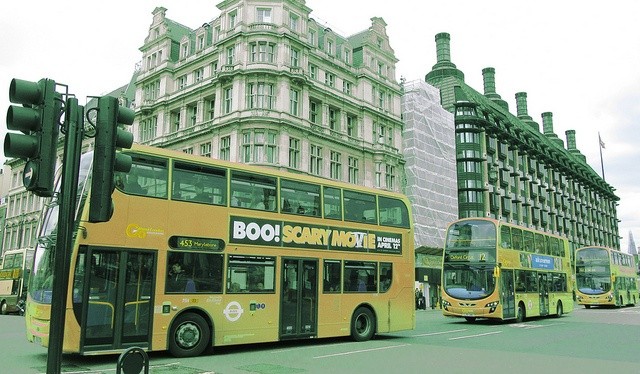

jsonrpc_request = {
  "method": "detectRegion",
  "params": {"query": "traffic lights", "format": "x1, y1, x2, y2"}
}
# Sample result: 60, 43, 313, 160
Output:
4, 79, 55, 194
87, 96, 133, 223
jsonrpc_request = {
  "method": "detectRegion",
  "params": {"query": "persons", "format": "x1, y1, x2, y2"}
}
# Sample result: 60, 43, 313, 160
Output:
415, 287, 425, 310
190, 183, 212, 203
252, 195, 266, 210
228, 282, 242, 292
169, 263, 187, 292
123, 173, 147, 194
255, 282, 266, 292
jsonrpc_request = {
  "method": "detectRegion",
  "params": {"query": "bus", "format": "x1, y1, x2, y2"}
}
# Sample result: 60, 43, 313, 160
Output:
26, 143, 415, 358
441, 217, 573, 323
0, 248, 37, 315
575, 246, 639, 308
